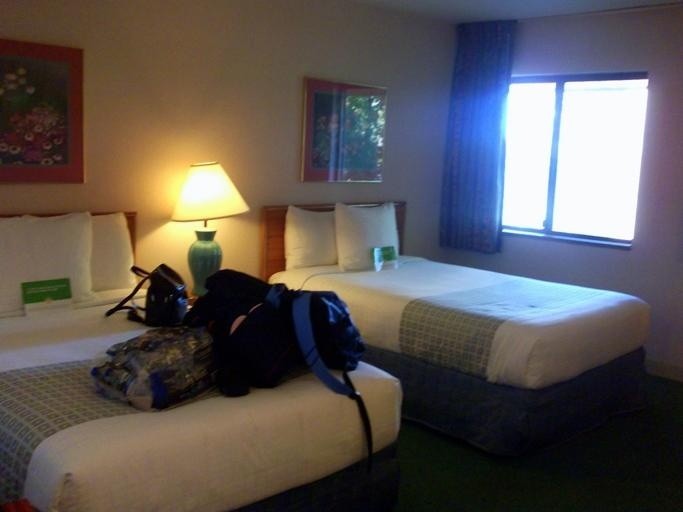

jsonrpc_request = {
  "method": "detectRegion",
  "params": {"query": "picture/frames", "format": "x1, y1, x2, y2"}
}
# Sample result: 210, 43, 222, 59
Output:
0, 38, 86, 183
301, 75, 390, 184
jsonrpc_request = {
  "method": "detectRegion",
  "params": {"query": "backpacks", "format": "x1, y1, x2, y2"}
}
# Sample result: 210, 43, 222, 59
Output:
103, 263, 190, 327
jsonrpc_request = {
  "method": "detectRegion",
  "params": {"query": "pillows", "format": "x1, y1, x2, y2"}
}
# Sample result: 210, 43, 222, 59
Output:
0, 210, 142, 315
282, 201, 402, 273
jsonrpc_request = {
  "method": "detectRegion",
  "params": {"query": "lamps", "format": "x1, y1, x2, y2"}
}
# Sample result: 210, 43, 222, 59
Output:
169, 161, 251, 297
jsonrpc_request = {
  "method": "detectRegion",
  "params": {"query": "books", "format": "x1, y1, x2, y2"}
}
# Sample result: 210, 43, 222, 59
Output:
370, 245, 399, 272
20, 278, 72, 312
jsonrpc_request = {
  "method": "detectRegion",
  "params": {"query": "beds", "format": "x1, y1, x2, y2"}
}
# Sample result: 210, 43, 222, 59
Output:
263, 201, 653, 456
0, 210, 404, 511
